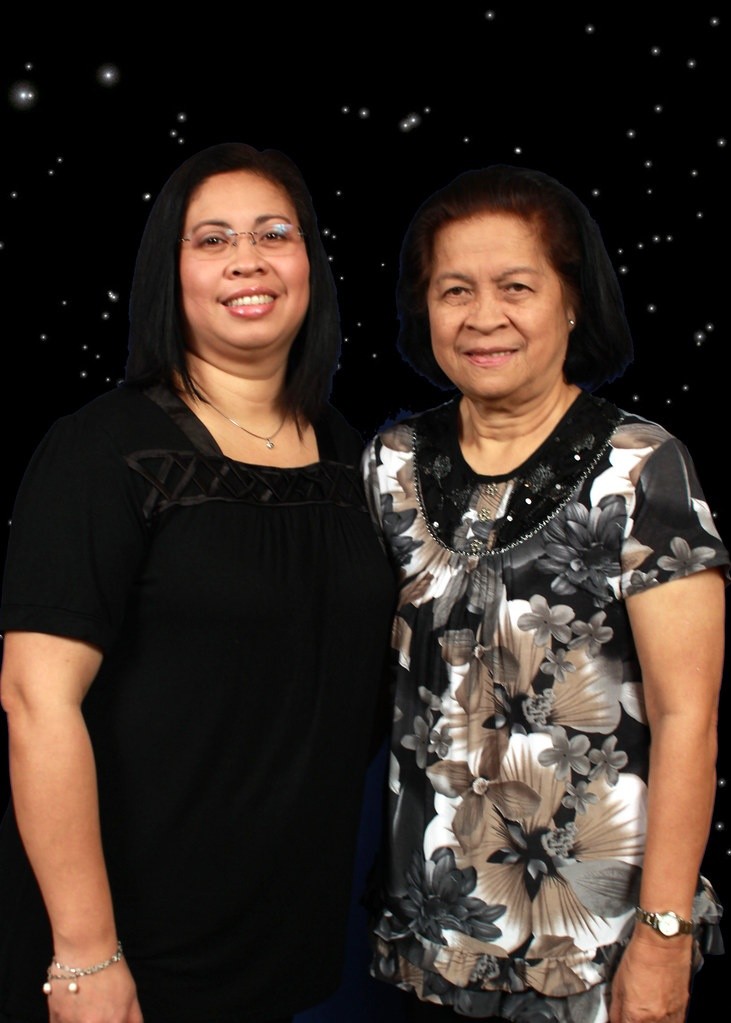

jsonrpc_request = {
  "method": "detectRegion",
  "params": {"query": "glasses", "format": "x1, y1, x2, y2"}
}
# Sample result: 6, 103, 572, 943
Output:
183, 225, 305, 255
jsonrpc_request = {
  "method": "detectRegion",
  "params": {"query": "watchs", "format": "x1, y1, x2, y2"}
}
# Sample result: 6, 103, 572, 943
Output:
635, 907, 697, 939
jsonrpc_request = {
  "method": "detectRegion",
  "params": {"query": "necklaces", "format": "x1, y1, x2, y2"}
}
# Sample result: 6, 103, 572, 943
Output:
204, 398, 288, 450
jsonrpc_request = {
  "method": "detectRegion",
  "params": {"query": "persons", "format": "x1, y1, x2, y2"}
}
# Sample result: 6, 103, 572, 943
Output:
360, 169, 731, 1023
0, 144, 403, 1023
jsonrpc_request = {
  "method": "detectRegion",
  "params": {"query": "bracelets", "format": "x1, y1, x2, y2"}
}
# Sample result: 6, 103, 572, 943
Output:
42, 939, 124, 996
